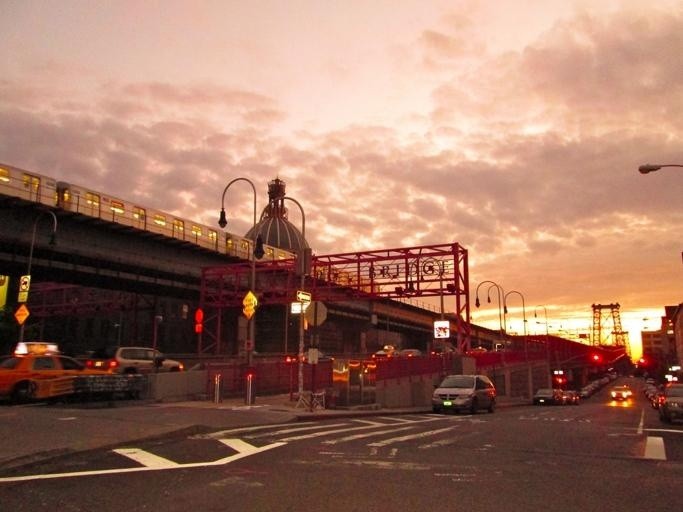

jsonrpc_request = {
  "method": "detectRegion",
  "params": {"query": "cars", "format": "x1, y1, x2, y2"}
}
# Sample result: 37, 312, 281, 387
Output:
282, 349, 336, 364
533, 373, 632, 405
370, 344, 459, 359
431, 374, 497, 414
643, 377, 683, 425
0, 342, 184, 403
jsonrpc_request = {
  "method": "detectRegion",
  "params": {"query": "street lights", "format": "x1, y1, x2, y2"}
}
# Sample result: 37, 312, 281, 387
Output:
476, 280, 549, 364
218, 176, 306, 396
19, 210, 59, 340
638, 164, 683, 175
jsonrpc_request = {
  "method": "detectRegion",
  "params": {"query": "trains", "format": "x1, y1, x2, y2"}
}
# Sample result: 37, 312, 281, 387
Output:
0, 159, 381, 297
